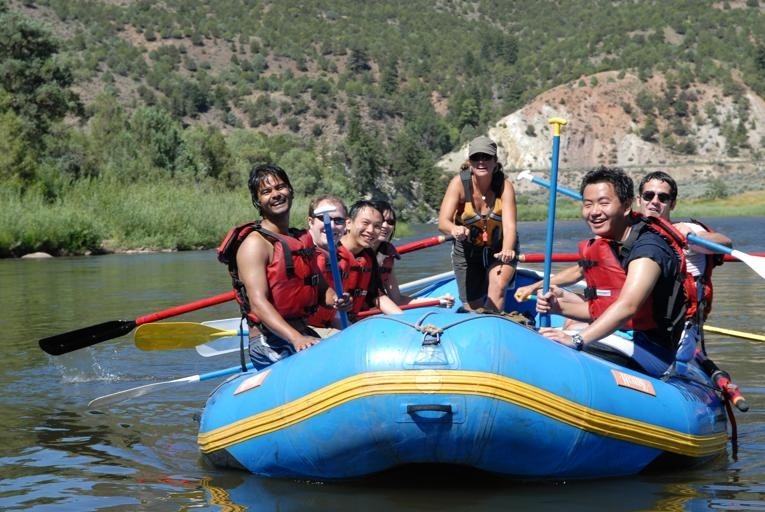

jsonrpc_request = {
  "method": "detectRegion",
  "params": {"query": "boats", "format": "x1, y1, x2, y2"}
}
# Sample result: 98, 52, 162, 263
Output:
194, 465, 716, 512
197, 266, 728, 484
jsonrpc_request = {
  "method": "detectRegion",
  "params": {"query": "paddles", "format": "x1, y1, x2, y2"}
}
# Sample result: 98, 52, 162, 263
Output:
87, 363, 254, 408
135, 299, 248, 351
194, 270, 455, 357
517, 168, 765, 284
516, 290, 765, 340
38, 230, 455, 355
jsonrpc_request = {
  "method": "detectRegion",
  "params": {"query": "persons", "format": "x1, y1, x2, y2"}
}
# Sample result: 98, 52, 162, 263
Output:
303, 193, 455, 339
216, 164, 354, 372
535, 164, 685, 379
436, 136, 520, 315
513, 172, 733, 362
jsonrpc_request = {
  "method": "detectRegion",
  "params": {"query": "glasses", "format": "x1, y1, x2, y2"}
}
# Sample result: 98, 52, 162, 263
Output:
641, 191, 671, 203
471, 154, 492, 161
314, 215, 345, 225
383, 219, 396, 225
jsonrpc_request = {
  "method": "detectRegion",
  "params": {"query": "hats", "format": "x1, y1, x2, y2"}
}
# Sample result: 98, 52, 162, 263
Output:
469, 137, 497, 157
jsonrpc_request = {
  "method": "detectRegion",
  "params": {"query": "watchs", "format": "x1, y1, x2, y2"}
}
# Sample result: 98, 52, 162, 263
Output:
571, 333, 584, 352
